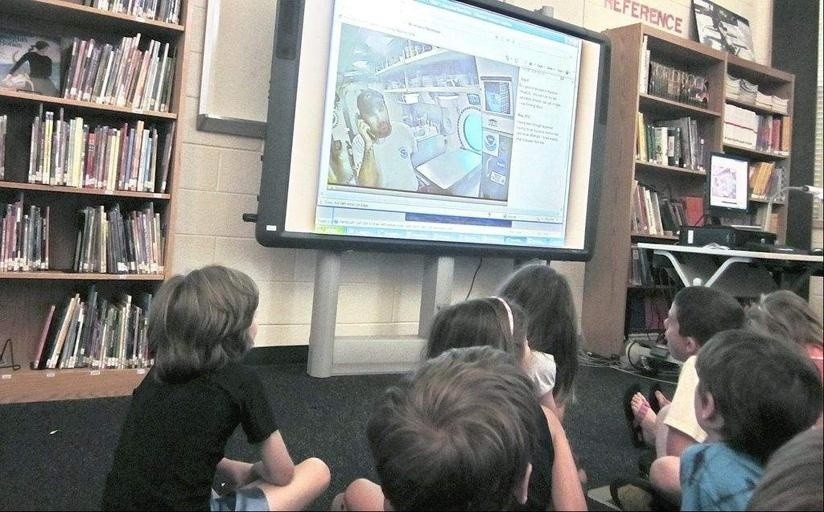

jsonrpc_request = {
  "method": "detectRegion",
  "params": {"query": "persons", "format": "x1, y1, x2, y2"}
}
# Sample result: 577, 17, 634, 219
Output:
649, 329, 823, 511
631, 290, 823, 447
333, 297, 590, 512
743, 428, 823, 511
367, 345, 541, 512
100, 265, 332, 512
353, 87, 419, 192
610, 287, 747, 512
499, 265, 579, 423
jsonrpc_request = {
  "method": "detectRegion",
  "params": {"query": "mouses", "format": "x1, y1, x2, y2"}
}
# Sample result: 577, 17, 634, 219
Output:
809, 248, 823, 255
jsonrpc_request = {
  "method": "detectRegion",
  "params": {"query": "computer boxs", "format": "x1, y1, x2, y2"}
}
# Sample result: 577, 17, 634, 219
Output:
680, 226, 776, 250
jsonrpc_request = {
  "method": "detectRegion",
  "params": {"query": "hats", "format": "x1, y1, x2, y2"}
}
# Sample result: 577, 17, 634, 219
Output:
357, 89, 384, 112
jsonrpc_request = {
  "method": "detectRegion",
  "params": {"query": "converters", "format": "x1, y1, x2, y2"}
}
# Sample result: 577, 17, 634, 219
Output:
649, 346, 669, 358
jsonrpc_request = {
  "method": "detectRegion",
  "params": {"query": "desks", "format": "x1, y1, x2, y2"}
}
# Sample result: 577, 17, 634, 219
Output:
624, 241, 824, 370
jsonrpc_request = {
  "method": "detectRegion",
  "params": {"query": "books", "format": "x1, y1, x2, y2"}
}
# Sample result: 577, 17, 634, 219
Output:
752, 203, 778, 242
76, 0, 184, 26
62, 33, 178, 114
638, 112, 704, 170
631, 178, 704, 235
749, 161, 787, 201
72, 201, 165, 276
27, 103, 176, 194
725, 75, 788, 113
724, 105, 790, 155
33, 284, 156, 371
0, 192, 49, 271
638, 35, 710, 109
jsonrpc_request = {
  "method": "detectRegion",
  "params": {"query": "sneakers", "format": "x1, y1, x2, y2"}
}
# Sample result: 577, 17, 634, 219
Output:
610, 478, 679, 510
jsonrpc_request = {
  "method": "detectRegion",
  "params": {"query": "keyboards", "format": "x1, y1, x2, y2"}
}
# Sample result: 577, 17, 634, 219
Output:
749, 247, 807, 255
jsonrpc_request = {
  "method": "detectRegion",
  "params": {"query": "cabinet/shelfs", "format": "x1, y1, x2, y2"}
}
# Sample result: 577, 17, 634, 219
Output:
581, 21, 798, 357
1, 0, 195, 405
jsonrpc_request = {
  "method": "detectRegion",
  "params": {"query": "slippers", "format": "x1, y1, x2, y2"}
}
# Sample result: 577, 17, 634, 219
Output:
623, 382, 662, 448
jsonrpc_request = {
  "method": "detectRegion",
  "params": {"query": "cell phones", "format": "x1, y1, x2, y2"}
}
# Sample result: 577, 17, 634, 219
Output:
358, 115, 375, 139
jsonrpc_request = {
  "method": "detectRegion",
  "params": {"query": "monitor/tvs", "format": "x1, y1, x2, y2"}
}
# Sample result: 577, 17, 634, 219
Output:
706, 151, 750, 225
